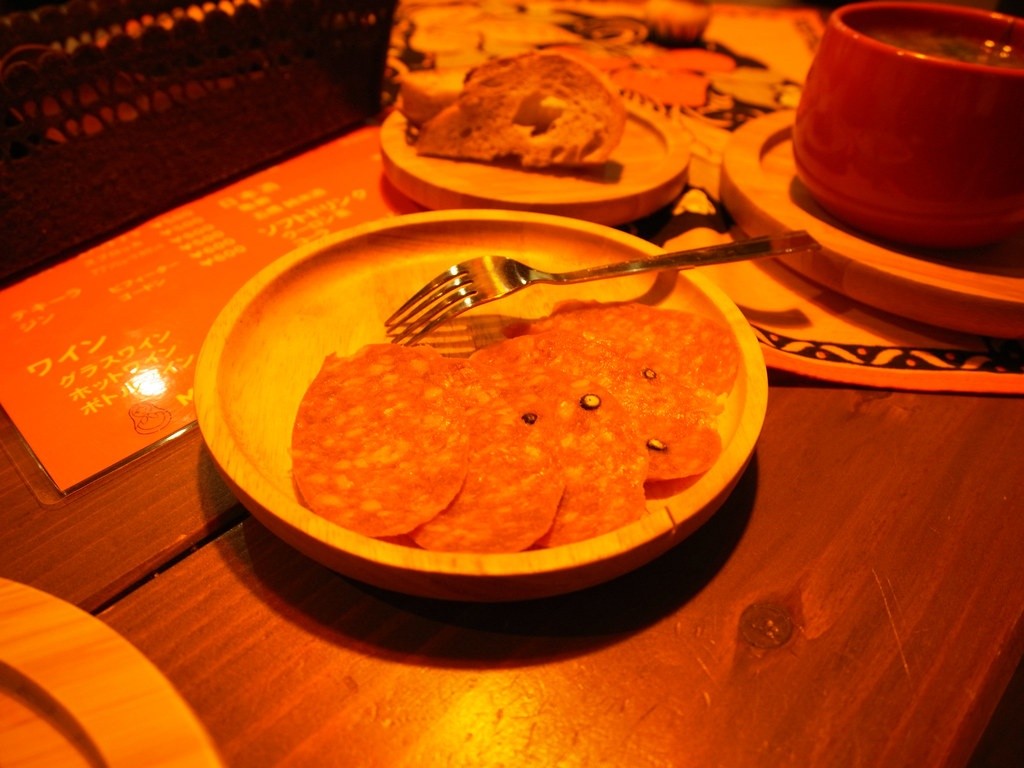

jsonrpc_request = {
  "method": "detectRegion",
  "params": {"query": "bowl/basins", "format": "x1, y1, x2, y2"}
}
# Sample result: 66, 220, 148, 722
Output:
795, 4, 1023, 248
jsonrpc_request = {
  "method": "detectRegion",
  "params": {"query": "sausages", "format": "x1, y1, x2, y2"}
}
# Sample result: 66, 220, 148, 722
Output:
293, 301, 738, 553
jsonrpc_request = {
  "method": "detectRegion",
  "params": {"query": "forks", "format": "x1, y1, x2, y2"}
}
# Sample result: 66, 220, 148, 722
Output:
384, 229, 822, 348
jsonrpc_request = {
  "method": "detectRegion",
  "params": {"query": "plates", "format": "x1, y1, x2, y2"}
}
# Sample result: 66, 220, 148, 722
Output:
1, 577, 223, 768
194, 209, 769, 600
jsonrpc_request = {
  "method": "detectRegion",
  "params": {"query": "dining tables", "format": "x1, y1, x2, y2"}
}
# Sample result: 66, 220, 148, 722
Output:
0, 120, 1024, 768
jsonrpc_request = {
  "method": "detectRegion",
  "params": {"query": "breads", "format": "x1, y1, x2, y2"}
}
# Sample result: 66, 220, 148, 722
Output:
399, 55, 627, 164
